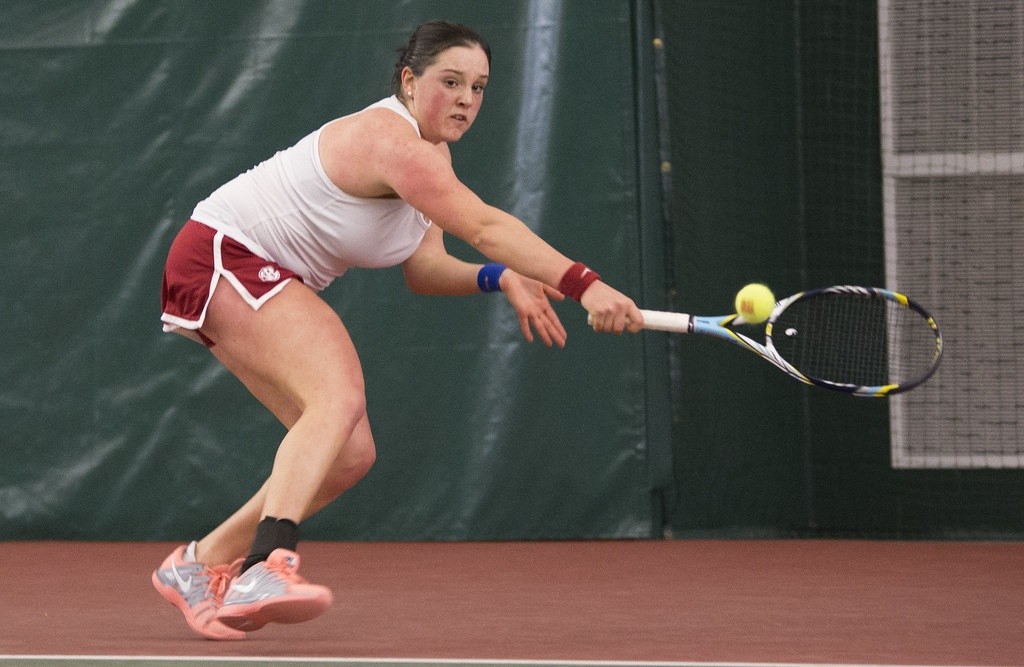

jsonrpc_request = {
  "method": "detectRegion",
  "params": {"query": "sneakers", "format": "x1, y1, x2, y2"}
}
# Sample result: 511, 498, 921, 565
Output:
151, 543, 246, 641
216, 548, 333, 632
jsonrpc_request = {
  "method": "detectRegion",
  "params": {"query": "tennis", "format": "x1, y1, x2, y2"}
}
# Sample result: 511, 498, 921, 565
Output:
735, 284, 776, 325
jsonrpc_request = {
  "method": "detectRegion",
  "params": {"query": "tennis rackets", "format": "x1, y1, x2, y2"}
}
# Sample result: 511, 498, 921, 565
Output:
587, 283, 945, 399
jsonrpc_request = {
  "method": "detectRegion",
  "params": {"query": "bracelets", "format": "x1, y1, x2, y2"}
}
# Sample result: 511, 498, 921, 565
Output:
556, 260, 603, 303
476, 264, 507, 294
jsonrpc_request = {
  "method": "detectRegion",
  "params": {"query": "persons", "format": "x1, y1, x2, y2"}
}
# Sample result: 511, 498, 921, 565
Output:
152, 23, 645, 643
